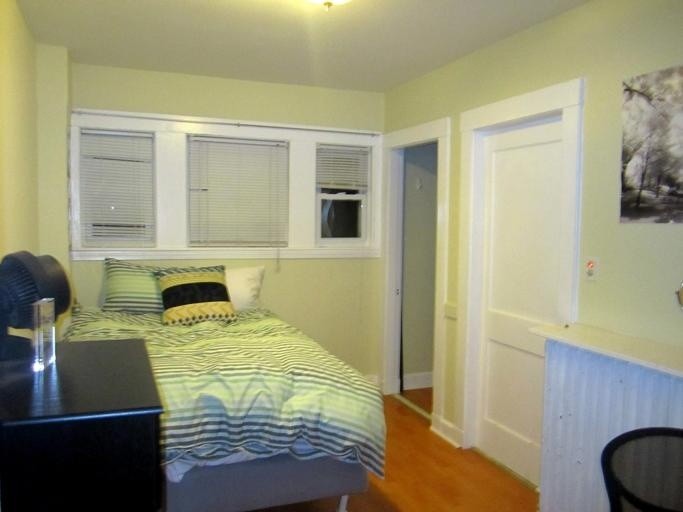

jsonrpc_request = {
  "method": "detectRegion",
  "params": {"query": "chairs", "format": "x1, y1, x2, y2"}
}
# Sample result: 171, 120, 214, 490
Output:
599, 426, 681, 512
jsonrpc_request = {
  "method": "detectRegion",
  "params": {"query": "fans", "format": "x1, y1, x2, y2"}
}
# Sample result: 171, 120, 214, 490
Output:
0, 250, 72, 350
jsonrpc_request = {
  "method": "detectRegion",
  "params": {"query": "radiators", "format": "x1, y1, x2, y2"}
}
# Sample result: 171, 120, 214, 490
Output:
542, 336, 682, 512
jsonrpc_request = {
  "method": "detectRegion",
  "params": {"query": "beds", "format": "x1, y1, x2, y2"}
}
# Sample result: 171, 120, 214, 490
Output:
59, 309, 387, 510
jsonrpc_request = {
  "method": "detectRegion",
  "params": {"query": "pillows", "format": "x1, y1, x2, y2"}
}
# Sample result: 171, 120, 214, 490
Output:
152, 264, 238, 327
224, 263, 268, 315
98, 256, 163, 314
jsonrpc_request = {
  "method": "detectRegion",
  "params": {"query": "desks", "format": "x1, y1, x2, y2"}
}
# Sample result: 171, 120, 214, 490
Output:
0, 335, 168, 511
